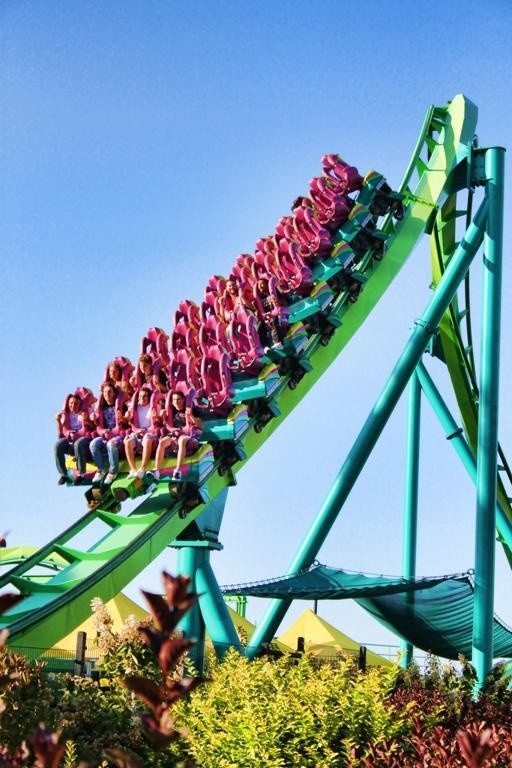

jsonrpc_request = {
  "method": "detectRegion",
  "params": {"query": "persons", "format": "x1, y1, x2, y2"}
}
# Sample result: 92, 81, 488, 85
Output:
216, 279, 255, 342
251, 278, 282, 354
52, 353, 202, 485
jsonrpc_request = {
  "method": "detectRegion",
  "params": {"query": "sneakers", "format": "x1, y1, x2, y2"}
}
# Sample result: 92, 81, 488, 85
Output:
54, 468, 184, 488
